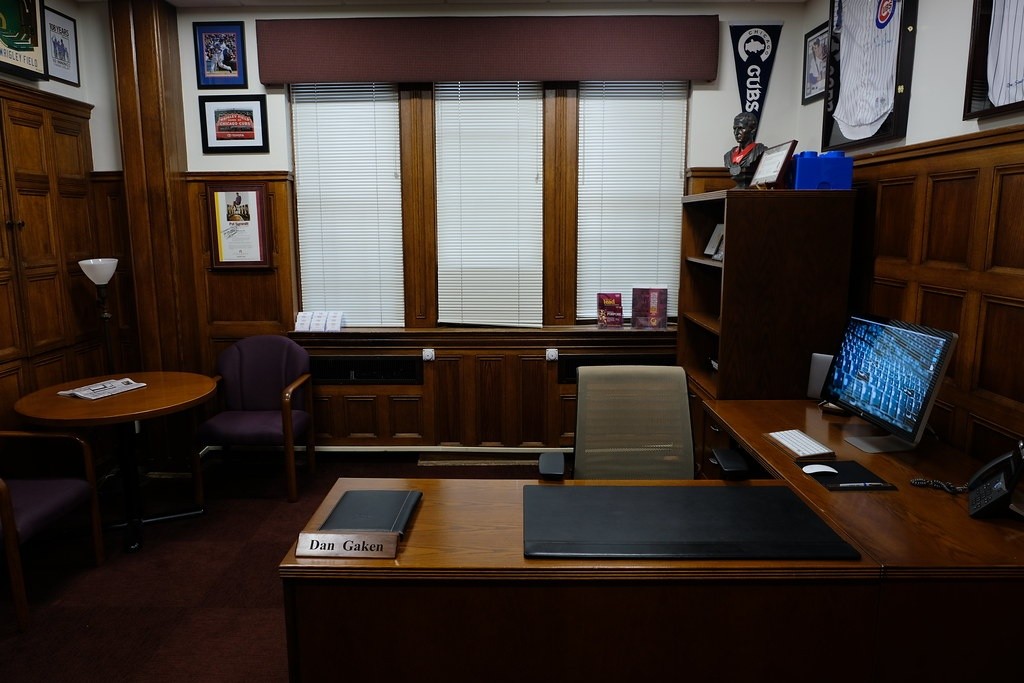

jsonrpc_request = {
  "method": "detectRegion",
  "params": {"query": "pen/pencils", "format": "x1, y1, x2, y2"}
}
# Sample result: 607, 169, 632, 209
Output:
839, 483, 884, 487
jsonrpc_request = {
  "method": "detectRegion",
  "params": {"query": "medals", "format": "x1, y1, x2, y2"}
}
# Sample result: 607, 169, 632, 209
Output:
730, 164, 741, 176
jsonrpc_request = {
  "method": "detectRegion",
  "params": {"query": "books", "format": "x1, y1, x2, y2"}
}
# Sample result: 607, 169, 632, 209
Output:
320, 489, 424, 537
632, 288, 669, 330
596, 293, 623, 330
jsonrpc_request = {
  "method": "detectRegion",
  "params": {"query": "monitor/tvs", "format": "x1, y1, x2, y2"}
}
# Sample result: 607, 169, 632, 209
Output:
820, 308, 958, 455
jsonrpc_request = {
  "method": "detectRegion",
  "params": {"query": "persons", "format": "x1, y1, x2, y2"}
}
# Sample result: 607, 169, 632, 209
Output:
724, 112, 770, 189
203, 33, 237, 73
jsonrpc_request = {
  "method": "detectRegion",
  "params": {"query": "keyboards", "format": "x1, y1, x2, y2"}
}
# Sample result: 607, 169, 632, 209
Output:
770, 426, 834, 458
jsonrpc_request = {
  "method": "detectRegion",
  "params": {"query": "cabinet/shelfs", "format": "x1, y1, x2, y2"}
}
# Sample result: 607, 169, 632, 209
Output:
701, 398, 1024, 683
677, 188, 857, 479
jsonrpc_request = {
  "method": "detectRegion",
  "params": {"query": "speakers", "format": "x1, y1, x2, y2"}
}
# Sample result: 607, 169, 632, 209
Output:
806, 352, 835, 399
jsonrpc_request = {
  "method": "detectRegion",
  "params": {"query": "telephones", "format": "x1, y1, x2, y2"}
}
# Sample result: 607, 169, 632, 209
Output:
967, 450, 1014, 517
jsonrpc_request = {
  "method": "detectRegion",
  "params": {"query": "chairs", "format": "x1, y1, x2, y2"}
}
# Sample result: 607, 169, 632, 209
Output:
0, 430, 106, 605
539, 366, 748, 479
189, 334, 312, 507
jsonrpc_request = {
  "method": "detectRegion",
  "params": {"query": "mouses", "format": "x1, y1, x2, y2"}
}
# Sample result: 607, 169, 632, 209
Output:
802, 463, 839, 476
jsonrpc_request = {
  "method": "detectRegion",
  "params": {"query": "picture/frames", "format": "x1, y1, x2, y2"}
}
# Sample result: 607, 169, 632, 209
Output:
0, 0, 81, 88
198, 94, 269, 153
801, 18, 829, 105
191, 20, 248, 90
205, 182, 275, 274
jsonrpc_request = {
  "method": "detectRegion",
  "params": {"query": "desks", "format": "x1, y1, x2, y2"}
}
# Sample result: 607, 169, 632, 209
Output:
278, 477, 881, 683
13, 371, 218, 554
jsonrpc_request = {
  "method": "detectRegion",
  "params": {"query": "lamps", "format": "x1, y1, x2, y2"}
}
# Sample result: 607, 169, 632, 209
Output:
78, 257, 119, 375
